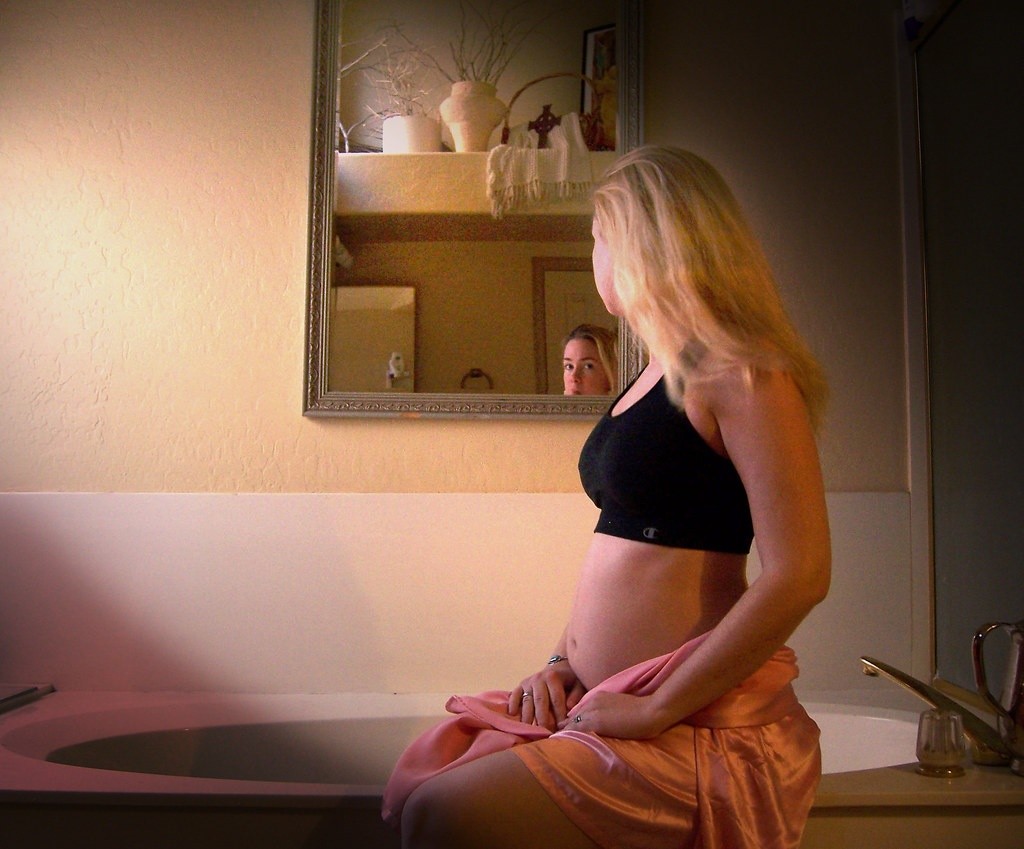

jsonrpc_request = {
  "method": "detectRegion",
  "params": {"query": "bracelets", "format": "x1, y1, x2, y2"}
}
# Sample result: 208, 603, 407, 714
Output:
548, 655, 567, 665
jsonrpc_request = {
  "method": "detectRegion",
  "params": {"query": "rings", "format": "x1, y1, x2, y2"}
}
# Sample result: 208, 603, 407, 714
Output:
577, 716, 581, 722
522, 692, 533, 697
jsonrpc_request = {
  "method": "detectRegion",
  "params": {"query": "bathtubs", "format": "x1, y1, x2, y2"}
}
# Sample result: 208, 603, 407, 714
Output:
0, 692, 1022, 849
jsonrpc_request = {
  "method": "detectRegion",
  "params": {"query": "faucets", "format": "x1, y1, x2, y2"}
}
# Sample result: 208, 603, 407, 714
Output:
859, 654, 1023, 776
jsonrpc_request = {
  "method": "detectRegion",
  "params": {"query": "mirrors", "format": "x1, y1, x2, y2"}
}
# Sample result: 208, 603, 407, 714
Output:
301, 0, 644, 423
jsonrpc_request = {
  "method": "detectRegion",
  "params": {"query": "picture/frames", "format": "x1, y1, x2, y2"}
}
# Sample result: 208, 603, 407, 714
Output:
579, 22, 619, 115
531, 256, 619, 395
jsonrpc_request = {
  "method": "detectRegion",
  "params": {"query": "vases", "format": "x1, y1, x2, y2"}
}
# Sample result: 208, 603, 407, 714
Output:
381, 116, 441, 153
439, 79, 508, 150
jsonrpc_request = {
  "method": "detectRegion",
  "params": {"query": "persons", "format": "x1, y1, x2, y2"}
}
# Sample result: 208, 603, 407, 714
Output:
562, 323, 618, 396
381, 144, 833, 849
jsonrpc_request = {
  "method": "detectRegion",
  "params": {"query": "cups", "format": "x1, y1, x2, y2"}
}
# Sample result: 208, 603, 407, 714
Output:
917, 709, 967, 777
